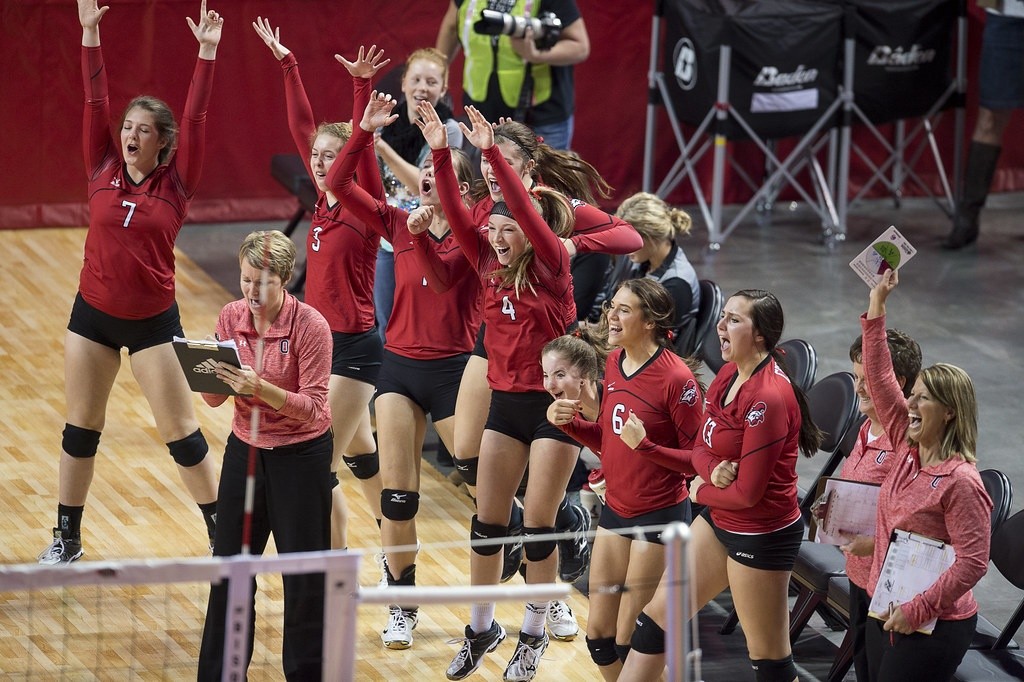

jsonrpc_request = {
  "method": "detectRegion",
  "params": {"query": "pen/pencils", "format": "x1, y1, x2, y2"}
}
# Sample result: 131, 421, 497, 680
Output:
215, 333, 219, 341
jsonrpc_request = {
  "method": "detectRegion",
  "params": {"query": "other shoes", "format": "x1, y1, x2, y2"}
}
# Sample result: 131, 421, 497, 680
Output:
448, 470, 470, 495
436, 438, 453, 467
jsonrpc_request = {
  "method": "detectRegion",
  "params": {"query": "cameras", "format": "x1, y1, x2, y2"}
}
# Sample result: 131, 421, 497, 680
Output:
472, 8, 563, 51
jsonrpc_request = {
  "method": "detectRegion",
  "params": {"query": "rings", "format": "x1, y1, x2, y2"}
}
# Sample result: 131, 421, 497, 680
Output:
232, 381, 235, 386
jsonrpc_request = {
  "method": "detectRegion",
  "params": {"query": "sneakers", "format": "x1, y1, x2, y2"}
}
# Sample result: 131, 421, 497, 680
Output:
545, 598, 580, 640
556, 505, 592, 583
38, 527, 84, 566
499, 506, 524, 583
374, 539, 420, 589
444, 618, 507, 681
382, 601, 419, 649
503, 628, 549, 682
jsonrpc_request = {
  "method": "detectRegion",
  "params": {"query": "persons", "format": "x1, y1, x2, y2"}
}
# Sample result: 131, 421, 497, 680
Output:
434, 0, 589, 150
938, 0, 1024, 251
547, 278, 709, 682
616, 288, 830, 682
36, 0, 225, 564
858, 268, 995, 682
197, 232, 333, 682
839, 327, 923, 682
252, 15, 700, 682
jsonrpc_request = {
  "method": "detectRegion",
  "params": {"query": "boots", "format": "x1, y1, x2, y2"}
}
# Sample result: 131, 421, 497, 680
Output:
930, 139, 1001, 250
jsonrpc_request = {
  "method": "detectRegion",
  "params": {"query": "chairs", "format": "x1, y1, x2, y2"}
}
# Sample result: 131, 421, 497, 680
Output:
698, 279, 1024, 682
